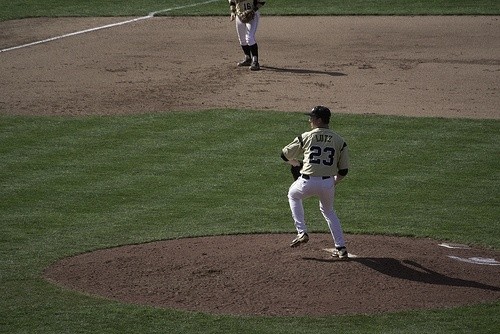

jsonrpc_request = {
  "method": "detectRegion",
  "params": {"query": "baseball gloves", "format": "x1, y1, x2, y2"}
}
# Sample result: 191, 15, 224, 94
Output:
238, 9, 254, 23
291, 160, 303, 181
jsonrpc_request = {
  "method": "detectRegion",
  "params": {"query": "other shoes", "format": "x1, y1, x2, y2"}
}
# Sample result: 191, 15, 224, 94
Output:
290, 233, 309, 248
332, 247, 347, 262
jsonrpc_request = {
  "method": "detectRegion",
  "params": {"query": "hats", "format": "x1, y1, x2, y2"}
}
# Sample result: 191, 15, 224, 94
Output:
304, 106, 331, 118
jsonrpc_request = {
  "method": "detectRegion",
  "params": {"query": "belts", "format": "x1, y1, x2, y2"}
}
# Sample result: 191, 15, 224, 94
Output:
302, 174, 330, 179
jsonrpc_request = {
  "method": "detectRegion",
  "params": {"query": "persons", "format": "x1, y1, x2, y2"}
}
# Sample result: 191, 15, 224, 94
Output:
281, 106, 348, 259
229, 0, 265, 70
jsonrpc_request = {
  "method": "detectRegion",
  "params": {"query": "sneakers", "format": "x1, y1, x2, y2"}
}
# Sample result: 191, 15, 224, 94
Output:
237, 60, 259, 71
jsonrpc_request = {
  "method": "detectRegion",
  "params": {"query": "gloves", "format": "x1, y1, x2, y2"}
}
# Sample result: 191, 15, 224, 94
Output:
230, 13, 236, 21
